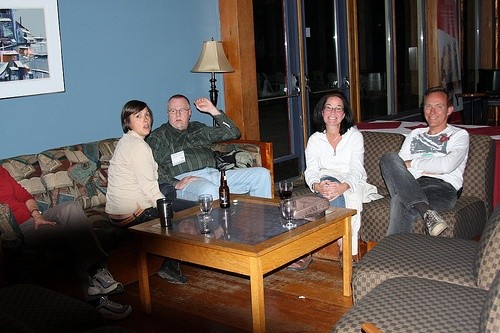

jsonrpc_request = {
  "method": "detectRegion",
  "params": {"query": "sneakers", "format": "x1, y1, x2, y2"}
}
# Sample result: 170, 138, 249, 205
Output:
423, 210, 449, 237
95, 295, 132, 322
157, 259, 187, 285
87, 268, 124, 298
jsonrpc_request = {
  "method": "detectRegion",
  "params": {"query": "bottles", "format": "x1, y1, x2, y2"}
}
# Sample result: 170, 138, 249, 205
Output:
220, 209, 231, 241
219, 170, 230, 208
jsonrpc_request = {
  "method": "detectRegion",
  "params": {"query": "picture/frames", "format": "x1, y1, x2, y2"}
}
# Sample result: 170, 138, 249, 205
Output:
0, 0, 66, 99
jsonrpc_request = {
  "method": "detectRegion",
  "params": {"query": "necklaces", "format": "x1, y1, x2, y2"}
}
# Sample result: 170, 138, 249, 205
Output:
332, 134, 340, 144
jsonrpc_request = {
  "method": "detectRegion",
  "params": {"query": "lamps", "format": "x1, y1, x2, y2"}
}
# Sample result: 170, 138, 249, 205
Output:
190, 37, 235, 126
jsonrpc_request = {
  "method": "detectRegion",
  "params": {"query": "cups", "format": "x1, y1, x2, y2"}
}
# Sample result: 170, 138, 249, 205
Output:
197, 212, 212, 234
281, 199, 296, 219
278, 180, 293, 199
156, 198, 174, 229
198, 194, 212, 215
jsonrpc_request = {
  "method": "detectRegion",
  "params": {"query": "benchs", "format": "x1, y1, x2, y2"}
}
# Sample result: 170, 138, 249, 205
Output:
0, 137, 275, 261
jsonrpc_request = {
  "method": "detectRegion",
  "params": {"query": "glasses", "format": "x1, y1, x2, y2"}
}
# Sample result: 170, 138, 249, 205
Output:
323, 105, 344, 112
422, 103, 447, 110
168, 108, 190, 114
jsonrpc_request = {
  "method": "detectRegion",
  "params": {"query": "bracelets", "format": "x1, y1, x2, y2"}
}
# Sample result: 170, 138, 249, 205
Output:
30, 208, 42, 216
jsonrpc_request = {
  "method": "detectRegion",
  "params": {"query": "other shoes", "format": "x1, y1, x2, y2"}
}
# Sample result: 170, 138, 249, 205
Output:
287, 253, 313, 272
338, 251, 358, 269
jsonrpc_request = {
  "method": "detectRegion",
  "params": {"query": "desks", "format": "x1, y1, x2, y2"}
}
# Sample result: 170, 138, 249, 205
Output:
455, 91, 500, 125
126, 195, 357, 333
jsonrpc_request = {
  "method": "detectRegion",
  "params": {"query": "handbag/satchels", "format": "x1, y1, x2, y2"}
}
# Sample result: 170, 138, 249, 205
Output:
277, 192, 330, 222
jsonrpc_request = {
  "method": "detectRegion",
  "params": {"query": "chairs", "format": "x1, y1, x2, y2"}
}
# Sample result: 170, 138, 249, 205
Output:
310, 131, 500, 333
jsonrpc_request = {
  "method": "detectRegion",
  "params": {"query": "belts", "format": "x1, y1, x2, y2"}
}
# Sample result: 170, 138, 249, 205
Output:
109, 208, 145, 227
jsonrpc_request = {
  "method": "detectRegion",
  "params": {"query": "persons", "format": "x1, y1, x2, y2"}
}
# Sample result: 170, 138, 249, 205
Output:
105, 100, 198, 284
287, 91, 367, 270
379, 87, 470, 236
145, 94, 273, 202
0, 165, 131, 320
